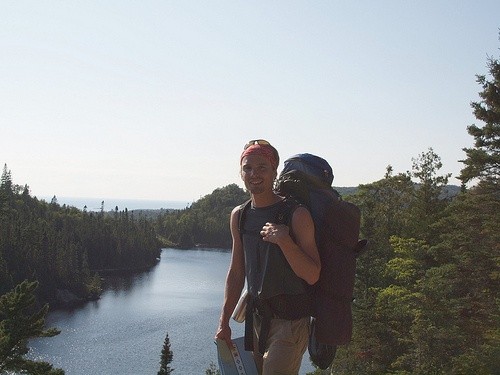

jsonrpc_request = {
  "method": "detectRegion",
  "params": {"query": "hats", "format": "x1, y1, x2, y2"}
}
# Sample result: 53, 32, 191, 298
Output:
240, 144, 280, 168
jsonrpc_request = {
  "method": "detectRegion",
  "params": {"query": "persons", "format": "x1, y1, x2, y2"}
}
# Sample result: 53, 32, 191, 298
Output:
214, 140, 322, 375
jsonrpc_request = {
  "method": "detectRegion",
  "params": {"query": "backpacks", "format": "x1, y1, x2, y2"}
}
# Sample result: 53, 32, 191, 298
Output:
236, 153, 367, 371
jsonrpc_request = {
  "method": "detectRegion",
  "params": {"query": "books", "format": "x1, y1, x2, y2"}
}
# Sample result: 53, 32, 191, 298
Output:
214, 336, 259, 375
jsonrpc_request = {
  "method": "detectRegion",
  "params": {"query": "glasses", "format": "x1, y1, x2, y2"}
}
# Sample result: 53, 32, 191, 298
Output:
243, 138, 270, 150
